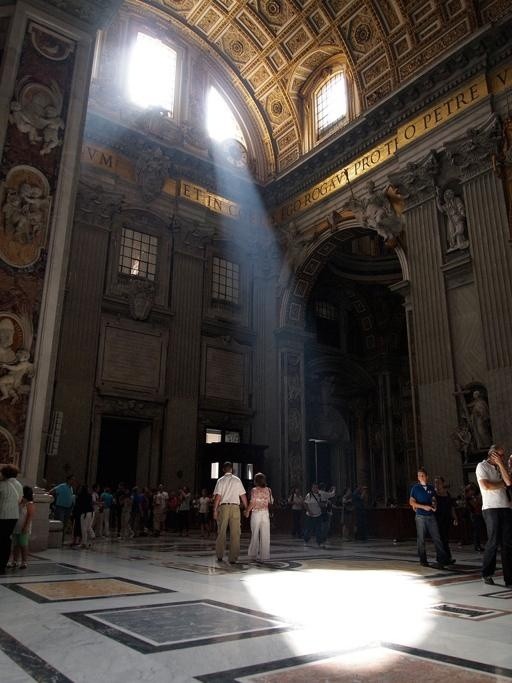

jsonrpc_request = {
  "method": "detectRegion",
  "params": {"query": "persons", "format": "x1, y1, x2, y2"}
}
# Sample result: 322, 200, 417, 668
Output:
288, 483, 374, 548
7, 485, 35, 569
408, 442, 512, 591
0, 348, 34, 405
7, 99, 42, 146
46, 475, 214, 549
246, 472, 274, 563
213, 461, 248, 564
39, 105, 65, 154
3, 182, 43, 246
272, 220, 317, 298
348, 180, 402, 241
0, 463, 24, 575
466, 390, 492, 450
435, 185, 470, 251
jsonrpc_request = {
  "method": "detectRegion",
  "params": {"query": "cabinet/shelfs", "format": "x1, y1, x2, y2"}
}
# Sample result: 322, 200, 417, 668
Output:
272, 505, 419, 543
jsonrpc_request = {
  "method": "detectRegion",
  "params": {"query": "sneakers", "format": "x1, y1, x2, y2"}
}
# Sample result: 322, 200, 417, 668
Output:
437, 559, 456, 566
7, 560, 18, 567
218, 557, 223, 561
20, 561, 28, 569
70, 528, 217, 548
482, 576, 495, 586
230, 561, 236, 564
419, 559, 429, 567
251, 555, 265, 564
291, 531, 369, 550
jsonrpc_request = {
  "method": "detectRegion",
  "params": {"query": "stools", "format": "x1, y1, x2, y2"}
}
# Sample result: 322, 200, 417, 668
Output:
49, 520, 64, 548
